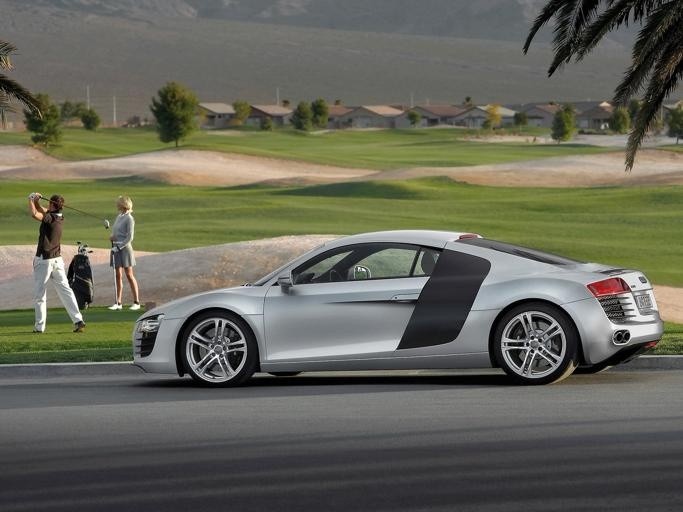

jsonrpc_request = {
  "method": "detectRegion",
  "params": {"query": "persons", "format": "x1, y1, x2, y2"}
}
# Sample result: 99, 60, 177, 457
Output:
26, 192, 86, 333
105, 196, 140, 310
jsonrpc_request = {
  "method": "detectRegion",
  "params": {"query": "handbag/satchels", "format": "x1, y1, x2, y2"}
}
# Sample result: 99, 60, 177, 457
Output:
67, 254, 93, 304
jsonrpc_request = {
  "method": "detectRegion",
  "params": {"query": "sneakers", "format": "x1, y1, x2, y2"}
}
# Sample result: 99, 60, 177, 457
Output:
128, 304, 140, 310
32, 329, 44, 333
72, 321, 85, 332
107, 304, 122, 311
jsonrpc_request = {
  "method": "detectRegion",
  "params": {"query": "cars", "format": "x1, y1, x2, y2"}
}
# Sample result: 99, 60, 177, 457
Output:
132, 230, 664, 386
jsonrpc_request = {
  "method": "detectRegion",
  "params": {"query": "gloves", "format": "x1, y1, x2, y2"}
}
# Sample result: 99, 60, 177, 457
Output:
103, 219, 109, 228
28, 192, 35, 199
111, 247, 119, 253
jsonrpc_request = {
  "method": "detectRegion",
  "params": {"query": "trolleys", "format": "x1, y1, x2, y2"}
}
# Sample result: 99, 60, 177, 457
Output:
67, 255, 94, 310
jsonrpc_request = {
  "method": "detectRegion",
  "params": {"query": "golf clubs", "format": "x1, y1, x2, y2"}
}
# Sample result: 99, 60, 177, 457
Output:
110, 240, 120, 309
76, 241, 94, 255
39, 196, 110, 229
